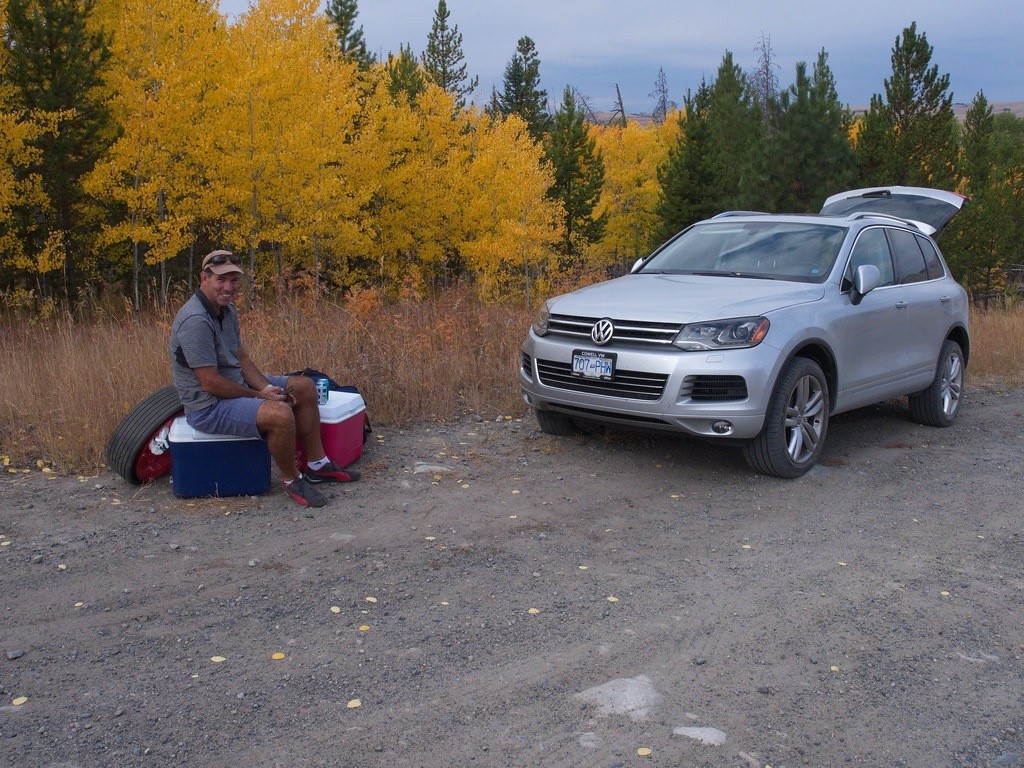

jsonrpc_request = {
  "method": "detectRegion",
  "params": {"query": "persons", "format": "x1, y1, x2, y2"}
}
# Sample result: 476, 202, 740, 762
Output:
169, 250, 362, 508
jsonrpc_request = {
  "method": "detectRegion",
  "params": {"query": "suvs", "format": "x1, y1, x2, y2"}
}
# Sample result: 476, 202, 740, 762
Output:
517, 186, 973, 479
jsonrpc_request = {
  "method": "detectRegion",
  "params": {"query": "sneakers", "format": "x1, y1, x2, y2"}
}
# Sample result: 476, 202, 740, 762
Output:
306, 460, 361, 482
280, 475, 327, 508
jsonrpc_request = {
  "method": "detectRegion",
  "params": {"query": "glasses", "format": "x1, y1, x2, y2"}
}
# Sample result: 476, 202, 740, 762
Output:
203, 254, 242, 269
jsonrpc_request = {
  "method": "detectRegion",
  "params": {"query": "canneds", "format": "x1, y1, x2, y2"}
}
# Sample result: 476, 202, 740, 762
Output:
315, 379, 329, 405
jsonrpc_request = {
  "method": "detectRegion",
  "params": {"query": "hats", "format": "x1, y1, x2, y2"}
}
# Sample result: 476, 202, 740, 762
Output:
202, 250, 245, 274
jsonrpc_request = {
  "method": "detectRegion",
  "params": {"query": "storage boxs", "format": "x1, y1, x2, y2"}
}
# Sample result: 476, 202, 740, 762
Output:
169, 414, 272, 499
295, 391, 367, 473
